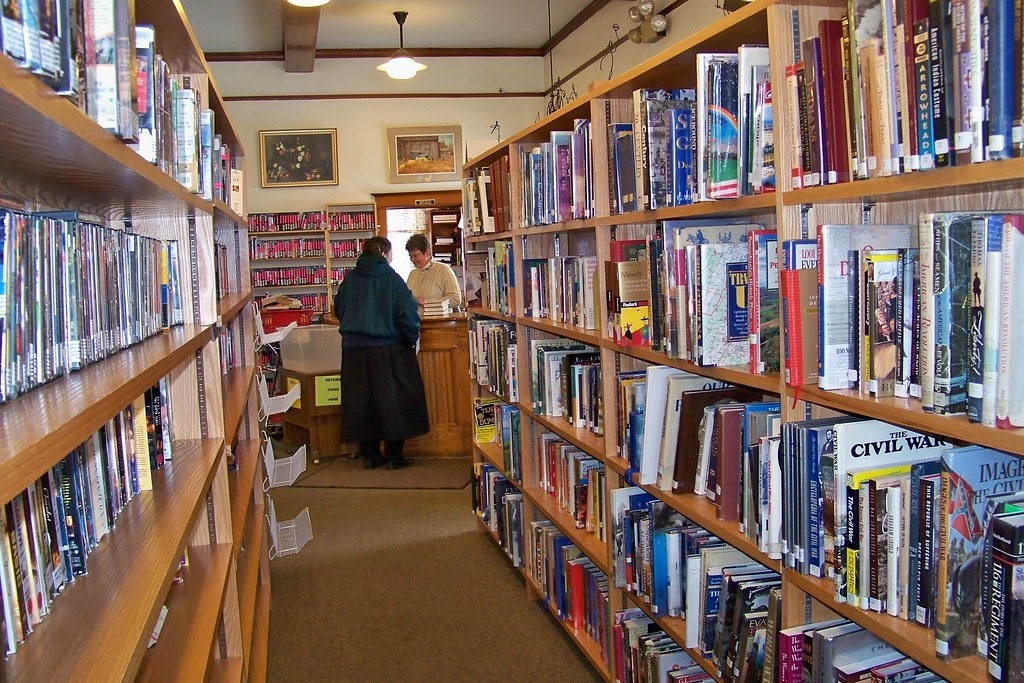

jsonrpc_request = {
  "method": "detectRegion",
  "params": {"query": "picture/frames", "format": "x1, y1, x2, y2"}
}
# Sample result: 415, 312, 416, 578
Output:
385, 124, 465, 184
258, 127, 339, 189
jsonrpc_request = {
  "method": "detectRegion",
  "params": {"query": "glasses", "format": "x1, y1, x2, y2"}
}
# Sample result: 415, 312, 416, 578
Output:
408, 248, 427, 259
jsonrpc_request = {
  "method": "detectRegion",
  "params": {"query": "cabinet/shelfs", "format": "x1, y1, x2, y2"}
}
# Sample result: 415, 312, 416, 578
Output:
1, 0, 1024, 683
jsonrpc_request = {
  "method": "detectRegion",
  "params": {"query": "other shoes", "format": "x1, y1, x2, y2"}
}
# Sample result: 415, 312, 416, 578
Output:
364, 452, 384, 470
384, 458, 412, 470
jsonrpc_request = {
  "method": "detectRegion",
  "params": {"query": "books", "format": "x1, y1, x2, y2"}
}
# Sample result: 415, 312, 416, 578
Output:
434, 248, 461, 267
0, 0, 245, 657
250, 210, 375, 312
434, 236, 454, 245
259, 347, 281, 369
423, 299, 450, 316
464, 0, 1024, 683
433, 215, 457, 223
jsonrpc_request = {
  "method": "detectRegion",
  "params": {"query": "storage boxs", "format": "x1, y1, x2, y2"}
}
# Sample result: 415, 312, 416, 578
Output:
275, 323, 343, 375
260, 308, 314, 333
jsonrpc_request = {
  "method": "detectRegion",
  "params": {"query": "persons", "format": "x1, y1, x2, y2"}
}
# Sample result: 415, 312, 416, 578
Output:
334, 236, 430, 470
404, 233, 461, 315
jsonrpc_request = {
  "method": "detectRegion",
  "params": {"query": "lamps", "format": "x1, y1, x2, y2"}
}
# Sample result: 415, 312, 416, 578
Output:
627, 0, 667, 44
376, 11, 427, 80
